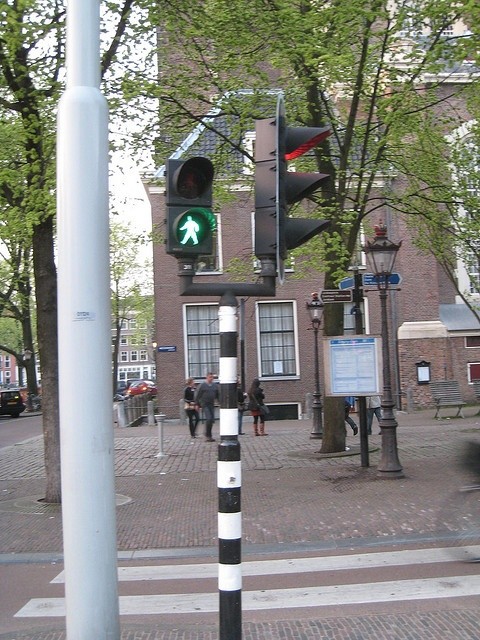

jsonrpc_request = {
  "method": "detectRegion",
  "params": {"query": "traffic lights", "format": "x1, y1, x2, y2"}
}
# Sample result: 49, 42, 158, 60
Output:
254, 95, 335, 286
165, 156, 215, 259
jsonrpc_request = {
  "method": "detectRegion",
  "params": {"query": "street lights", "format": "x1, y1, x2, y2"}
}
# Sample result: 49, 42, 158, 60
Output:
304, 291, 324, 443
361, 219, 405, 480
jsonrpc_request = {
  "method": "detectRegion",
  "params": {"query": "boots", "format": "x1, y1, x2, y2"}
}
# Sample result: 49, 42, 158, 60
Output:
260, 423, 268, 436
253, 424, 261, 436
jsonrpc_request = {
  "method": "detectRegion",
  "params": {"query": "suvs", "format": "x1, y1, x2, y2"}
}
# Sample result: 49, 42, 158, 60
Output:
0, 387, 26, 421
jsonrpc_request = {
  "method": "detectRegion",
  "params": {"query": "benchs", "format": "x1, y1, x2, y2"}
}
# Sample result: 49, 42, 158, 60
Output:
431, 381, 468, 420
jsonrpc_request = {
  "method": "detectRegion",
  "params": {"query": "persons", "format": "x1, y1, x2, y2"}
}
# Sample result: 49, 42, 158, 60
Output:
194, 371, 219, 442
365, 396, 383, 436
183, 377, 198, 439
343, 396, 358, 437
247, 378, 268, 437
237, 373, 248, 435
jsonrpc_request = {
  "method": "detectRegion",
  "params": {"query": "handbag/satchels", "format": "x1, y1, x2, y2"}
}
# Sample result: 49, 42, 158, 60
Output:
257, 404, 270, 415
184, 401, 199, 412
236, 401, 250, 411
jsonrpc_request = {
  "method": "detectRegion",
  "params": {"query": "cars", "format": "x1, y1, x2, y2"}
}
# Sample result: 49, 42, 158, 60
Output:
126, 381, 157, 398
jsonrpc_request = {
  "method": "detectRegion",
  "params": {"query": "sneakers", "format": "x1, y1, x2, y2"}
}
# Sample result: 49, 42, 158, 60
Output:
210, 438, 215, 442
205, 436, 210, 442
353, 425, 358, 435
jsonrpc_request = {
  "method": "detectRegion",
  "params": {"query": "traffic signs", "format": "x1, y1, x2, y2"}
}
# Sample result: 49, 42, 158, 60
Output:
323, 334, 384, 397
